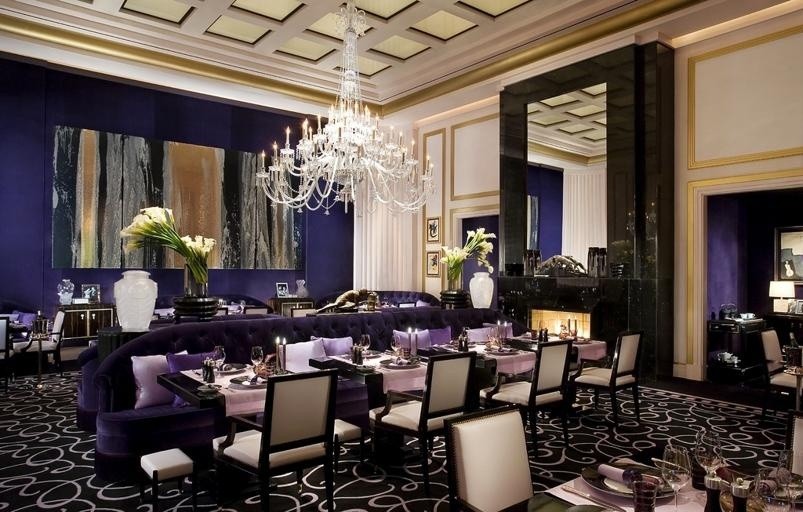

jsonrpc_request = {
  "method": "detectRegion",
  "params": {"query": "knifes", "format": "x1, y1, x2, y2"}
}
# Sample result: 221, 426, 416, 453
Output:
561, 484, 626, 512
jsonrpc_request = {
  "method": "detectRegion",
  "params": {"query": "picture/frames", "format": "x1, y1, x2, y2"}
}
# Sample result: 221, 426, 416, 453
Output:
425, 216, 441, 244
276, 282, 289, 299
424, 249, 444, 277
773, 225, 803, 286
795, 300, 803, 315
81, 283, 101, 305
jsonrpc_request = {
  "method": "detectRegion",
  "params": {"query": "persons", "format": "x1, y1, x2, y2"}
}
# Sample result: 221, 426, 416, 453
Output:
314, 288, 366, 312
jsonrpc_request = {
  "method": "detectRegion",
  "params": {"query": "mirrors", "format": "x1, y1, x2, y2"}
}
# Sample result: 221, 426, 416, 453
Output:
525, 83, 607, 278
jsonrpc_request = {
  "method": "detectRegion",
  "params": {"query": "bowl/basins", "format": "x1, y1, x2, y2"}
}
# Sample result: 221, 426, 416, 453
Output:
505, 264, 524, 276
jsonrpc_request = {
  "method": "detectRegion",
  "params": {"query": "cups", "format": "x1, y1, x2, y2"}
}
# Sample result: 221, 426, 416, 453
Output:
740, 313, 747, 319
610, 262, 626, 279
747, 312, 756, 318
785, 346, 803, 374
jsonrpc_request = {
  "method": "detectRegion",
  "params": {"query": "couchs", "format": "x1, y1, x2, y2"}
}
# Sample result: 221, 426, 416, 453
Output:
315, 291, 441, 309
155, 294, 272, 314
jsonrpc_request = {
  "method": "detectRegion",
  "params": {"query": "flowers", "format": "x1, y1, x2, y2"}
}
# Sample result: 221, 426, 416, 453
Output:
120, 206, 216, 284
439, 228, 496, 280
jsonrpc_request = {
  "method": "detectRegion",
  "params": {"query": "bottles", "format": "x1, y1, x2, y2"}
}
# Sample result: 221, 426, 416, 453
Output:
543, 328, 548, 342
533, 249, 541, 267
351, 343, 358, 364
703, 470, 723, 512
598, 247, 608, 279
206, 358, 215, 383
202, 356, 212, 381
458, 336, 463, 351
587, 247, 599, 278
789, 331, 800, 349
531, 330, 537, 340
463, 337, 468, 352
630, 475, 659, 512
539, 330, 542, 341
403, 348, 411, 359
731, 477, 749, 512
523, 250, 534, 277
355, 343, 363, 364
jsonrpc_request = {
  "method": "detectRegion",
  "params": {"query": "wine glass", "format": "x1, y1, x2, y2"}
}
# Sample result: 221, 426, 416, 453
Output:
239, 300, 246, 310
360, 335, 370, 354
251, 345, 263, 375
696, 431, 722, 480
661, 444, 692, 512
720, 303, 731, 321
776, 450, 803, 512
487, 327, 497, 346
390, 335, 402, 358
729, 303, 738, 320
213, 345, 226, 377
383, 297, 389, 304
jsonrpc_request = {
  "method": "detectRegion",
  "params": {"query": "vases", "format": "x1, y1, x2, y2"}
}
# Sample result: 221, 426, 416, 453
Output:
57, 279, 75, 305
469, 272, 494, 309
295, 279, 309, 298
184, 257, 208, 297
447, 265, 462, 290
113, 269, 159, 332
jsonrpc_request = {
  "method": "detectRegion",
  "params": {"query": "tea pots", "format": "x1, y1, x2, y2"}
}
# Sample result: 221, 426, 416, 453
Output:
719, 351, 733, 363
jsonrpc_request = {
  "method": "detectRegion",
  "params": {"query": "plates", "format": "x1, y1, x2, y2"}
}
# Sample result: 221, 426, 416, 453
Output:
197, 384, 222, 394
485, 351, 519, 355
755, 473, 803, 504
565, 505, 607, 511
214, 362, 247, 374
230, 376, 267, 389
379, 359, 419, 368
582, 462, 681, 500
573, 340, 588, 345
361, 349, 382, 358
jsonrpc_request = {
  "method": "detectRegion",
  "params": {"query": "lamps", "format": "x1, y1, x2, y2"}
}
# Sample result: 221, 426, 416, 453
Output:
256, 0, 438, 217
769, 281, 795, 313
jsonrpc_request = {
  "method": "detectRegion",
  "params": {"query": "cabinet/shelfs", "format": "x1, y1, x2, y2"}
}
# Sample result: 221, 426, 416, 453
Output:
267, 298, 315, 318
